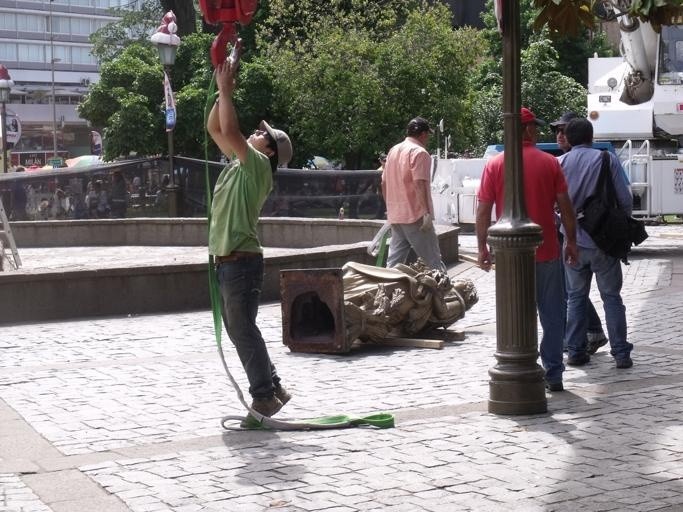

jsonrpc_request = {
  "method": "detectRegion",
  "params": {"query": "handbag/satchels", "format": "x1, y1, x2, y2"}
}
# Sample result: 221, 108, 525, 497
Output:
577, 151, 638, 265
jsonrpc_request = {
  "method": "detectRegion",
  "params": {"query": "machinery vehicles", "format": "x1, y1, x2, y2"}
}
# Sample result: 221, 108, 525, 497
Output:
584, 0, 682, 227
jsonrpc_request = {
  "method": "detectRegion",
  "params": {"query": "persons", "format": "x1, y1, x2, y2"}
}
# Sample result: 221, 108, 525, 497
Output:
379, 115, 445, 274
473, 107, 581, 393
204, 60, 292, 429
325, 263, 484, 347
7, 153, 171, 218
549, 113, 610, 357
556, 115, 635, 369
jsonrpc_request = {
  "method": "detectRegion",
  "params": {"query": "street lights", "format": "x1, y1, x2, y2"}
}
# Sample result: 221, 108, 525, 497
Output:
150, 8, 181, 216
49, 56, 62, 168
0, 63, 15, 172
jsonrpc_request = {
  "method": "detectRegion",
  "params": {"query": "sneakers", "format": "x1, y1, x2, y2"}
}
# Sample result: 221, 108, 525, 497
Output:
274, 385, 291, 404
240, 396, 283, 427
617, 352, 632, 368
567, 354, 589, 365
587, 338, 608, 355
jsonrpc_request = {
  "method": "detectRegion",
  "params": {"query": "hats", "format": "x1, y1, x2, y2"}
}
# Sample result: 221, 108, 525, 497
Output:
259, 120, 293, 165
408, 116, 435, 135
521, 107, 546, 126
550, 112, 577, 131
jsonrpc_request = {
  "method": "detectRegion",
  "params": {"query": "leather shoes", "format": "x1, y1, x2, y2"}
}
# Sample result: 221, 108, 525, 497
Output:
545, 382, 563, 391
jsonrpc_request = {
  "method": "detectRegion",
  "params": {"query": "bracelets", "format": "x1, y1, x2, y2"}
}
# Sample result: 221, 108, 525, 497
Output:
214, 98, 219, 106
